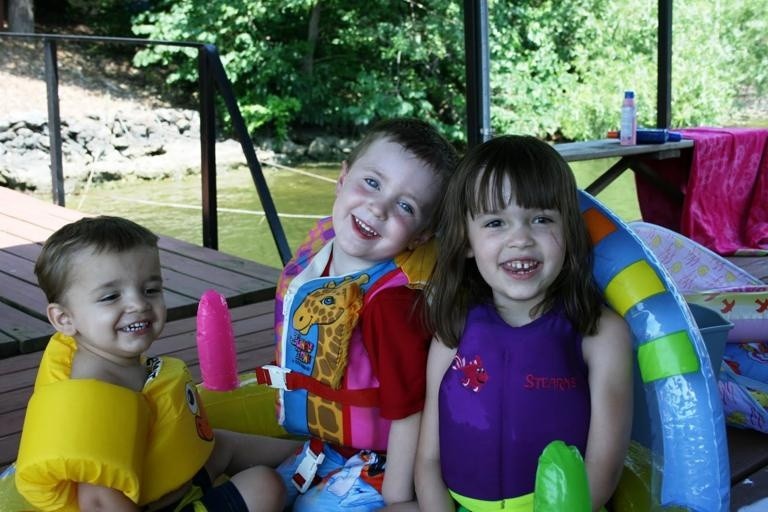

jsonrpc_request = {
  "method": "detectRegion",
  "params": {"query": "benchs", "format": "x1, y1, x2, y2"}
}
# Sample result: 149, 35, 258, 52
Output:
554, 137, 692, 203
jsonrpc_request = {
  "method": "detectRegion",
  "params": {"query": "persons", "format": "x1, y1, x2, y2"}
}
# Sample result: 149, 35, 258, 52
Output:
255, 110, 464, 512
372, 133, 638, 512
15, 214, 308, 511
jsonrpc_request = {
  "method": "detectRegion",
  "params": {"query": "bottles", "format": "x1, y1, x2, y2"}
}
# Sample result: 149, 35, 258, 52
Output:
618, 90, 638, 146
